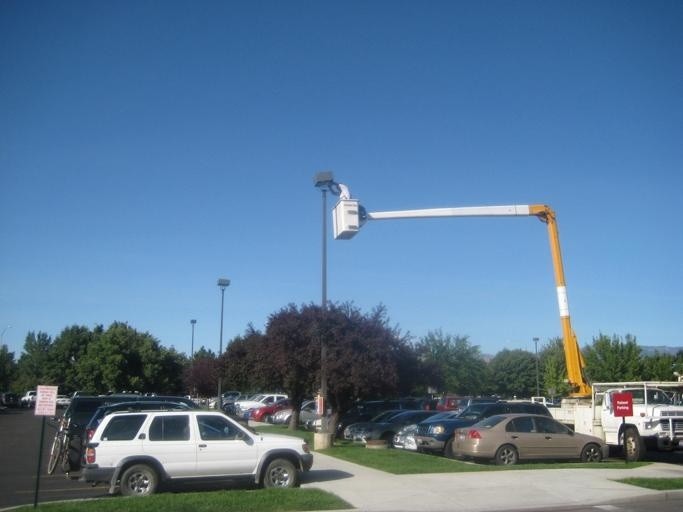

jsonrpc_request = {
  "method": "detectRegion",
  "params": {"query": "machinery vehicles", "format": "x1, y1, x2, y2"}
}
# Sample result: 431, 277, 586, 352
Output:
333, 198, 683, 461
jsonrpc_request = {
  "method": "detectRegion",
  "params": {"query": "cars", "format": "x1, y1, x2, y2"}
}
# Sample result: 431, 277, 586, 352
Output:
2, 390, 71, 408
338, 395, 610, 463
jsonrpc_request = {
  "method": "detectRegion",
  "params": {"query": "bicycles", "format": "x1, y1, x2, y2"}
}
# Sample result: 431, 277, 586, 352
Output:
48, 417, 82, 473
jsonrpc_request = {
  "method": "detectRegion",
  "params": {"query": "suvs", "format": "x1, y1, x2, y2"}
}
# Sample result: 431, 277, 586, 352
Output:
65, 390, 313, 497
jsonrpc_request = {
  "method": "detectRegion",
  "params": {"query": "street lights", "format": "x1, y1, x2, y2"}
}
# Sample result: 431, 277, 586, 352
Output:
190, 319, 199, 393
533, 337, 540, 396
315, 170, 333, 434
217, 278, 230, 410
